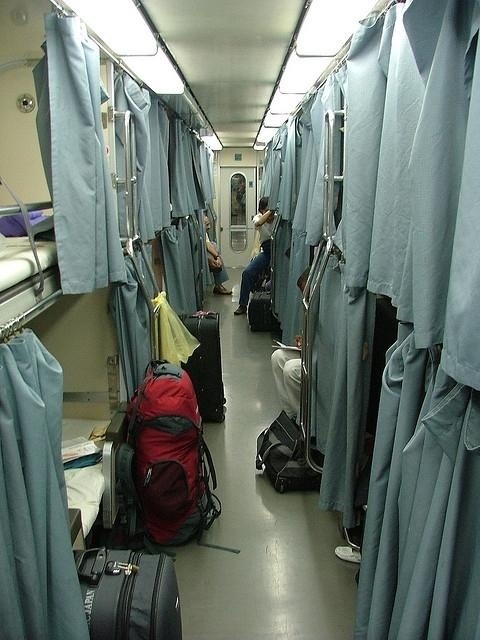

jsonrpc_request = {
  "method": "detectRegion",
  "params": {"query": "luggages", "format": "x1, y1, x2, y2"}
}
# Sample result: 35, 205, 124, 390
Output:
176, 309, 228, 425
249, 290, 278, 332
72, 548, 180, 638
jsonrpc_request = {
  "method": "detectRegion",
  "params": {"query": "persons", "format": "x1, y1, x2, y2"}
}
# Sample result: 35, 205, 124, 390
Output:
233, 196, 278, 316
201, 215, 233, 296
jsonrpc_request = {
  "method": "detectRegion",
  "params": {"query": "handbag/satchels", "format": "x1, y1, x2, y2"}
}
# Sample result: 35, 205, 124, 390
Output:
261, 239, 271, 256
118, 361, 211, 549
256, 411, 317, 492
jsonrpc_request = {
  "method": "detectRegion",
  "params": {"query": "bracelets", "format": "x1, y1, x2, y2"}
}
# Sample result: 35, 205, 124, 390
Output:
215, 255, 220, 259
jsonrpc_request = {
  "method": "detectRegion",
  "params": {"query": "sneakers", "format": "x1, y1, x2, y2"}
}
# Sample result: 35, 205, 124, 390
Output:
234, 305, 246, 314
335, 546, 362, 563
212, 285, 233, 296
